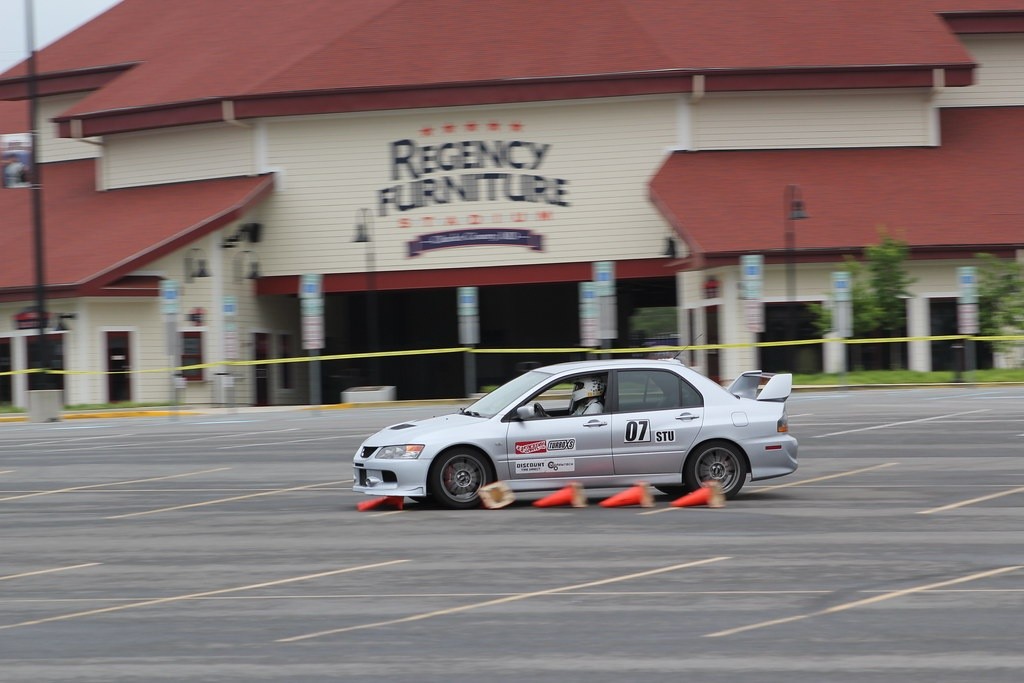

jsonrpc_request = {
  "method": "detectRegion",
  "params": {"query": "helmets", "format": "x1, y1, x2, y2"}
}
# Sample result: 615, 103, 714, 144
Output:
570, 374, 606, 403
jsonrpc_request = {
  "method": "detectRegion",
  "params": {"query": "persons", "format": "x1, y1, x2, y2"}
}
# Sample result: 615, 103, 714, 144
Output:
535, 377, 606, 418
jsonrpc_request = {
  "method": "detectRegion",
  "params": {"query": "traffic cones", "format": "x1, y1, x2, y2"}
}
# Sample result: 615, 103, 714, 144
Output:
597, 481, 655, 508
670, 481, 728, 507
356, 496, 404, 512
477, 479, 517, 509
532, 483, 586, 509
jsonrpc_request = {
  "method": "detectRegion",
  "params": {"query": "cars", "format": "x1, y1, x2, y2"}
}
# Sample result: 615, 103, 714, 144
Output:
352, 354, 800, 512
